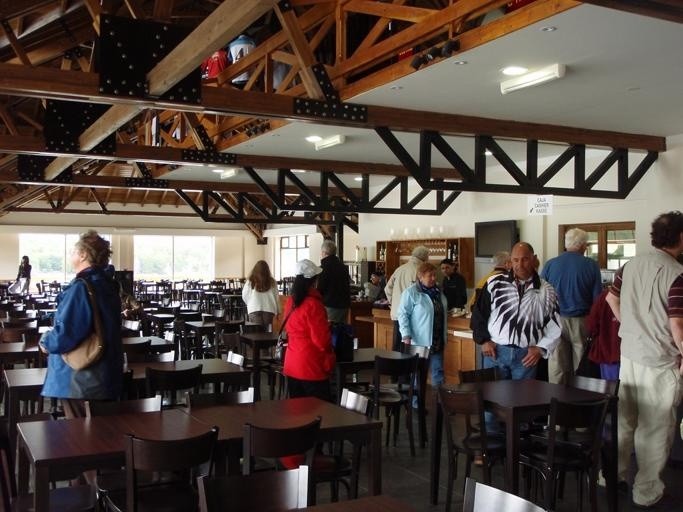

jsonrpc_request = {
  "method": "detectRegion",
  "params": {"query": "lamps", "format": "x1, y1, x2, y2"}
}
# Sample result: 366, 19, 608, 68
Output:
500, 62, 564, 98
315, 134, 345, 153
221, 168, 240, 183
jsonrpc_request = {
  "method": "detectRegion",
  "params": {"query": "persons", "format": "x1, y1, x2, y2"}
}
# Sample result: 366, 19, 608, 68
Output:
604, 210, 682, 507
470, 243, 562, 465
320, 242, 349, 321
384, 246, 448, 415
200, 30, 295, 90
540, 228, 620, 435
13, 255, 32, 294
276, 259, 335, 400
241, 260, 282, 331
439, 259, 468, 310
370, 272, 386, 303
39, 229, 128, 417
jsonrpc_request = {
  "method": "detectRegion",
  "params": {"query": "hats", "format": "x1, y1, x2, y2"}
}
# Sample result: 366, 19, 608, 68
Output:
295, 258, 325, 281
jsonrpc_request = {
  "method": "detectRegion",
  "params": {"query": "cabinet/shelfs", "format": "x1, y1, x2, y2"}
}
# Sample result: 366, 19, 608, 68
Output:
376, 237, 474, 289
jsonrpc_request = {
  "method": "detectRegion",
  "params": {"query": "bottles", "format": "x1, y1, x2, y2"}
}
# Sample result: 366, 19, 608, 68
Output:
379, 244, 383, 261
357, 290, 364, 298
447, 242, 457, 261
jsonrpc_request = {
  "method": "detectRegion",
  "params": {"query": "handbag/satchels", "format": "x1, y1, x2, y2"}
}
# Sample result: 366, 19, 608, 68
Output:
60, 275, 105, 373
273, 329, 285, 362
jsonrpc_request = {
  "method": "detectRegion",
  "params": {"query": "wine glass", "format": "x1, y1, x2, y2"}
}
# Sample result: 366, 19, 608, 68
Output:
394, 241, 445, 256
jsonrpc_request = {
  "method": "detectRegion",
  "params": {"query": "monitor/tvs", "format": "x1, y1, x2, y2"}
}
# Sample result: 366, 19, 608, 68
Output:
475, 220, 520, 257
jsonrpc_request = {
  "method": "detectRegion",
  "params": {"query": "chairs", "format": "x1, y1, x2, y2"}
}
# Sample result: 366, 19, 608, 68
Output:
2, 274, 623, 512
